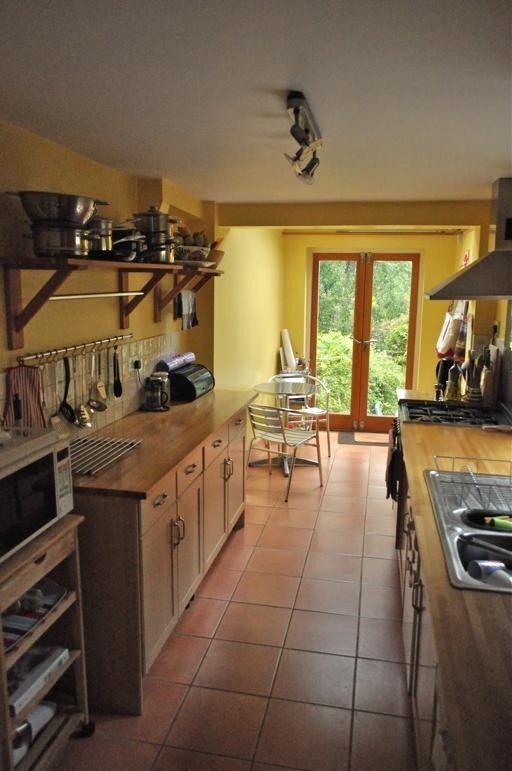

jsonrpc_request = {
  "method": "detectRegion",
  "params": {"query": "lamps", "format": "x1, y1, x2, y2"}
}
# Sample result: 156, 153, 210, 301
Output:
281, 94, 323, 184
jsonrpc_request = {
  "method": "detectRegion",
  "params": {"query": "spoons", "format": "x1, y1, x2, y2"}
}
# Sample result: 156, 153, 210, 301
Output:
60, 352, 111, 427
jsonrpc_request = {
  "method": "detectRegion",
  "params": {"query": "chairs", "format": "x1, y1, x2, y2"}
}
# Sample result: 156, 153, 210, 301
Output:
246, 403, 323, 501
268, 374, 330, 458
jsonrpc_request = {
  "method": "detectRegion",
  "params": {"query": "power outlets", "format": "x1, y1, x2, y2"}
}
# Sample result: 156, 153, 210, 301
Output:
128, 356, 140, 372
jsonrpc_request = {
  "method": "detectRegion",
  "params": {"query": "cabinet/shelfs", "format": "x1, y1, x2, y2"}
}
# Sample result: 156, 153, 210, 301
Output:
0, 515, 95, 771
74, 448, 200, 675
397, 486, 454, 771
203, 408, 246, 567
4, 255, 225, 351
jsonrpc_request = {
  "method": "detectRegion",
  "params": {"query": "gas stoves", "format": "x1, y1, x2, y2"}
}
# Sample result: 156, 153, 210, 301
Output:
402, 399, 507, 426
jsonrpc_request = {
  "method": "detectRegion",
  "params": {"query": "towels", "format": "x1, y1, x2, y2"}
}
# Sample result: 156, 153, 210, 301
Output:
179, 290, 199, 331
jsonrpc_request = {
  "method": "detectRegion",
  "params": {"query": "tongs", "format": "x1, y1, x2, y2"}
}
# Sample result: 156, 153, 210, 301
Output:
112, 352, 123, 398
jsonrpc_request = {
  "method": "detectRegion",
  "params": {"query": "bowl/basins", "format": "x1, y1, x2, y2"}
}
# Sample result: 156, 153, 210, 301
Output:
178, 245, 211, 261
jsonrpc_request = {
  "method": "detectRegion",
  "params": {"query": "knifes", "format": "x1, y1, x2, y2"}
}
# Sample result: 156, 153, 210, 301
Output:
482, 346, 491, 368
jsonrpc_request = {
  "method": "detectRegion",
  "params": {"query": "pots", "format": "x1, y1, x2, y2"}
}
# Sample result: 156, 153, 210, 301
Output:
3, 188, 181, 266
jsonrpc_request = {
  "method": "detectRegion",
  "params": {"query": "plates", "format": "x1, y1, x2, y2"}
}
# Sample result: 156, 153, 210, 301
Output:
175, 259, 216, 268
139, 405, 170, 412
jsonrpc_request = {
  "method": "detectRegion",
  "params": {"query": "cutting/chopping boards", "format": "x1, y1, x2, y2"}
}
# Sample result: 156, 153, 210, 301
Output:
489, 343, 502, 403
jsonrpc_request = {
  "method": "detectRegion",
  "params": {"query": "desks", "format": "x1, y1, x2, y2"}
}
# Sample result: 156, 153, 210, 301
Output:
250, 382, 319, 477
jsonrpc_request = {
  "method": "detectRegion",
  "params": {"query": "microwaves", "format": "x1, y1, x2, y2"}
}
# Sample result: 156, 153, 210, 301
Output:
0, 425, 76, 566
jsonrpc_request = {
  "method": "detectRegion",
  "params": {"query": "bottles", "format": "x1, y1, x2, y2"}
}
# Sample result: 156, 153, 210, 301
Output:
143, 370, 171, 408
434, 380, 454, 402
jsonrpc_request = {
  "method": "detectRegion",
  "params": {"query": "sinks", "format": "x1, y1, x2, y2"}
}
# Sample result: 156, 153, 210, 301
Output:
444, 530, 512, 594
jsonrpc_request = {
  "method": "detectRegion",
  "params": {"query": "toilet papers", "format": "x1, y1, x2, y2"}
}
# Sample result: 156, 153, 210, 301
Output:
157, 352, 196, 371
280, 328, 297, 371
